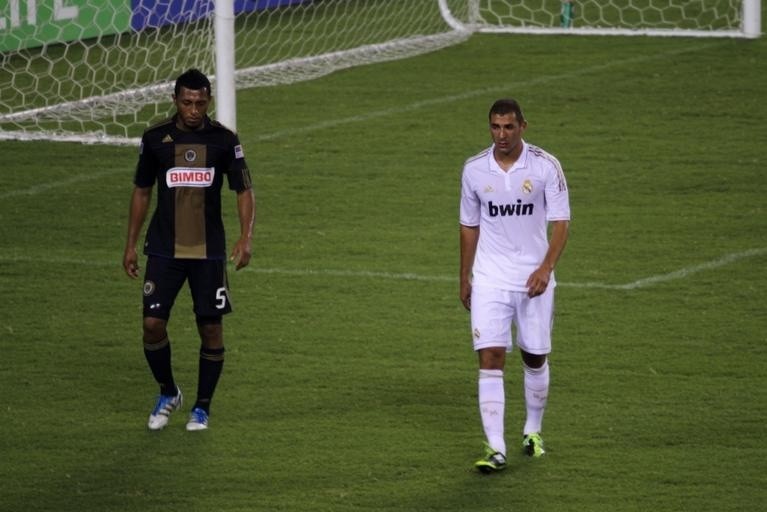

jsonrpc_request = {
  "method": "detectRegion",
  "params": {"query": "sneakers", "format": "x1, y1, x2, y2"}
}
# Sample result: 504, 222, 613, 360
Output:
522, 433, 546, 459
185, 407, 208, 431
148, 386, 184, 431
474, 440, 508, 474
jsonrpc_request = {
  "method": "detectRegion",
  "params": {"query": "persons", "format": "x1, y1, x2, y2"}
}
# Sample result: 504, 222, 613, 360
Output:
120, 67, 258, 434
458, 98, 571, 473
